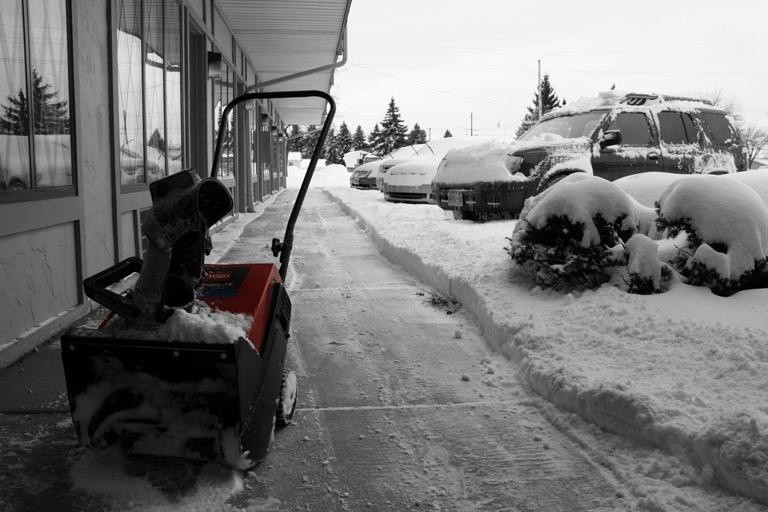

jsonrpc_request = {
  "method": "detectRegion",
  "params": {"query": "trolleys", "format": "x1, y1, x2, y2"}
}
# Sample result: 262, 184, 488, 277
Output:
61, 90, 337, 470
